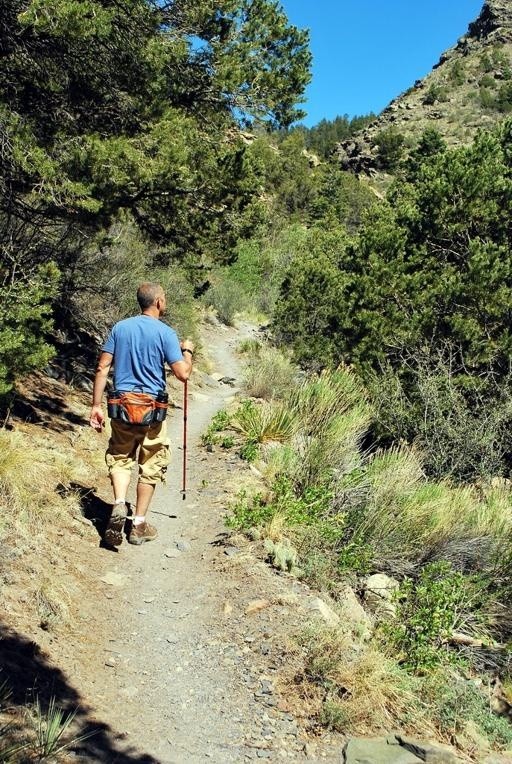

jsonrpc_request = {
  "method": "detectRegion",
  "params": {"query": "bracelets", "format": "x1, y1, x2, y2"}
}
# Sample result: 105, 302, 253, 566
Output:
182, 349, 194, 357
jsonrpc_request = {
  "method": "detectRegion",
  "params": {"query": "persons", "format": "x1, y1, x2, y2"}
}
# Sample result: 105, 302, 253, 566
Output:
90, 283, 194, 546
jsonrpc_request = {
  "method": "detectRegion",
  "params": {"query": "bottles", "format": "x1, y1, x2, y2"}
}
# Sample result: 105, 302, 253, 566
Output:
155, 392, 168, 422
107, 389, 121, 418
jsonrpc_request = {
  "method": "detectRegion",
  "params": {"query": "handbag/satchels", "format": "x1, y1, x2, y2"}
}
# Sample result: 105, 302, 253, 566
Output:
107, 389, 169, 426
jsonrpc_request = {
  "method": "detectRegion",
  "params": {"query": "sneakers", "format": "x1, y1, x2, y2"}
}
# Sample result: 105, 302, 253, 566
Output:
128, 521, 158, 544
104, 502, 128, 546
